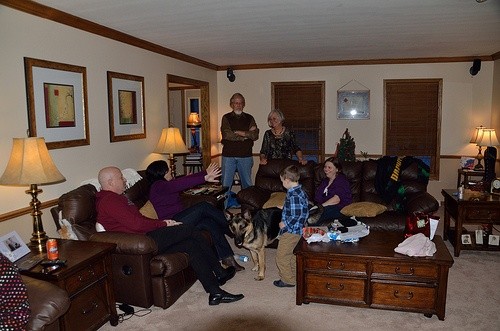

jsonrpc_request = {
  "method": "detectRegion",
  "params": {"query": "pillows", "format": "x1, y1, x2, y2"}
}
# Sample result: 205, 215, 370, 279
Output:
339, 201, 388, 217
262, 192, 286, 209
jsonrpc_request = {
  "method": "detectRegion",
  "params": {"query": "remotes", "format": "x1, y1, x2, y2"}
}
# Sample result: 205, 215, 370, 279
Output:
40, 259, 66, 266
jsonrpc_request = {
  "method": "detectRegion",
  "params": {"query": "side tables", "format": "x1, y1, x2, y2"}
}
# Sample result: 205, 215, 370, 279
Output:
14, 238, 119, 331
183, 184, 229, 208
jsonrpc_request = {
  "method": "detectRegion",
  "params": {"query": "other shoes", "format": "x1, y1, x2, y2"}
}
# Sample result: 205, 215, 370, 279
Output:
273, 280, 295, 287
223, 256, 245, 271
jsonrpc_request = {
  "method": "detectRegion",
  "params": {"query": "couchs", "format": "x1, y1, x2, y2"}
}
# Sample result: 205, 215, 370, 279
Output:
50, 169, 220, 310
234, 159, 439, 250
20, 275, 71, 331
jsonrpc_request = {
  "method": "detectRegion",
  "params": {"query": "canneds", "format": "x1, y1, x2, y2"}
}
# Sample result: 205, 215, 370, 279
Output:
458, 187, 464, 199
46, 239, 59, 260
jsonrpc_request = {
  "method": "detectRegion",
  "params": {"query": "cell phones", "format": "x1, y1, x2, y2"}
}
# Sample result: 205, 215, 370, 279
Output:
39, 264, 60, 275
204, 190, 214, 195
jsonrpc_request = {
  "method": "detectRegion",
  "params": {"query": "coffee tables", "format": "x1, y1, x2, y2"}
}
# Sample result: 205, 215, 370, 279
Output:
293, 233, 455, 321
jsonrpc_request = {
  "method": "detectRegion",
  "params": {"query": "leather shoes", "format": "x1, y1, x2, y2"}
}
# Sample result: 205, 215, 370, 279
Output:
216, 266, 237, 284
208, 290, 244, 305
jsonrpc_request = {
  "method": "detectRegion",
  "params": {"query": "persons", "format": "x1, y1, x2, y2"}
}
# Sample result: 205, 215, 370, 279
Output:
308, 157, 352, 227
274, 165, 310, 288
259, 109, 308, 165
221, 93, 259, 192
95, 166, 245, 305
147, 160, 245, 271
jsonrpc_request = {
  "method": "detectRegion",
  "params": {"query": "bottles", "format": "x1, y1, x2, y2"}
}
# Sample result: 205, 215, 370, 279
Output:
233, 253, 252, 263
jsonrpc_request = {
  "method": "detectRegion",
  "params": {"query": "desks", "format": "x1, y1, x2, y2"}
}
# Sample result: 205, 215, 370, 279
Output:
457, 169, 485, 189
441, 188, 500, 257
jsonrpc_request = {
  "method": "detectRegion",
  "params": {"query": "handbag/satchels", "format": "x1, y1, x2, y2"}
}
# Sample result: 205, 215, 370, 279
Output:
403, 211, 430, 242
428, 218, 440, 240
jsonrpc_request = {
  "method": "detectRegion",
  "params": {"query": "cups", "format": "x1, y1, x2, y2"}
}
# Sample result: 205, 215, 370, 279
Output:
458, 185, 465, 201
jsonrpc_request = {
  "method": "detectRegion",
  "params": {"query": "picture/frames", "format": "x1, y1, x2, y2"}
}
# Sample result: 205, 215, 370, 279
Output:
0, 231, 31, 263
107, 71, 147, 143
24, 57, 90, 150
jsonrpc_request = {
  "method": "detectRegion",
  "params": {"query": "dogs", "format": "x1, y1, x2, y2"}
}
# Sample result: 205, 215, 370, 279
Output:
224, 207, 283, 281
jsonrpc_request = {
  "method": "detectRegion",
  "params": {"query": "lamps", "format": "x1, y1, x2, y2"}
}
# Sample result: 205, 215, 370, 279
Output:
187, 112, 200, 153
476, 129, 500, 180
469, 59, 482, 76
227, 67, 235, 82
157, 127, 190, 180
469, 126, 487, 170
0, 137, 67, 250
